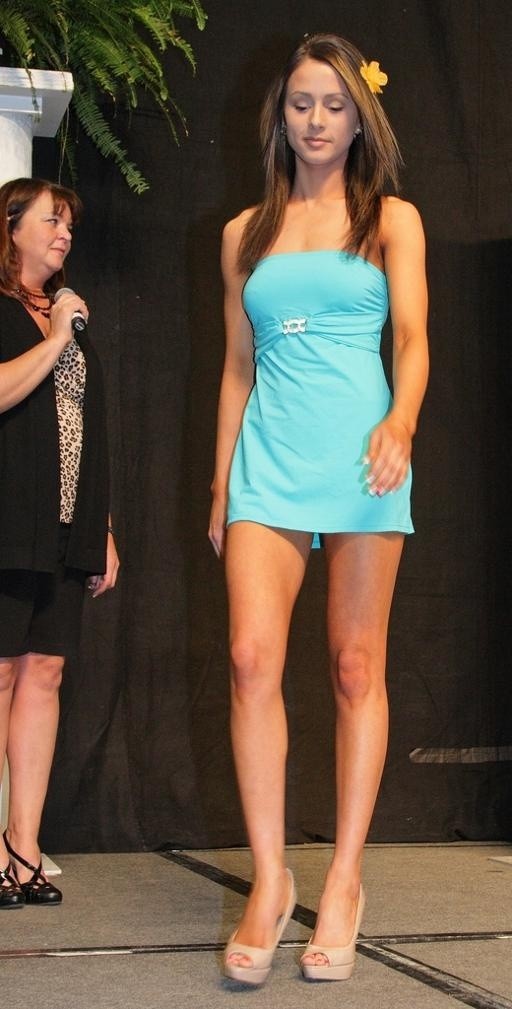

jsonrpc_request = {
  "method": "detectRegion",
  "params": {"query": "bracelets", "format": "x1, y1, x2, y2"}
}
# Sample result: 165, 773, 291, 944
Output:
107, 526, 116, 537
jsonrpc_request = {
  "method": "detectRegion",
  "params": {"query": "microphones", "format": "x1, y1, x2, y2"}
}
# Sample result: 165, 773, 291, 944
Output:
55, 286, 87, 332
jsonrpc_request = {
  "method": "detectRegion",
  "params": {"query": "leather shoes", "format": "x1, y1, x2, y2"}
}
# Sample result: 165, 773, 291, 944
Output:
0, 827, 63, 908
297, 869, 367, 980
220, 865, 300, 984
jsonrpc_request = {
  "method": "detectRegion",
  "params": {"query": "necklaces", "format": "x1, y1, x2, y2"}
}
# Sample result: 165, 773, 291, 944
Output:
17, 286, 55, 320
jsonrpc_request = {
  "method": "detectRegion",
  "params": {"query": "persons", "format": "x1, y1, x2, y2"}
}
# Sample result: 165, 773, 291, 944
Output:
1, 176, 123, 911
201, 31, 432, 991
